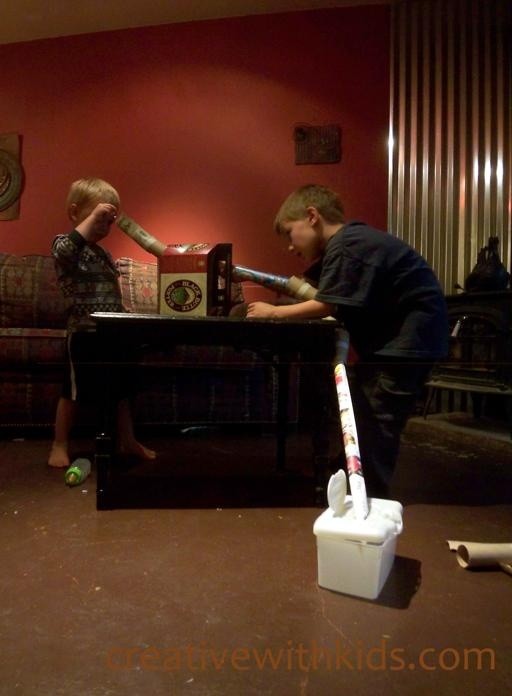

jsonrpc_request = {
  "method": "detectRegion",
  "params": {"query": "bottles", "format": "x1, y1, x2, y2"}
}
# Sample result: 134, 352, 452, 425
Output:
63, 457, 92, 487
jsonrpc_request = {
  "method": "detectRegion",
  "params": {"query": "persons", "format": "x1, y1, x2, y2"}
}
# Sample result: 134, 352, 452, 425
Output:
47, 178, 157, 468
247, 184, 451, 498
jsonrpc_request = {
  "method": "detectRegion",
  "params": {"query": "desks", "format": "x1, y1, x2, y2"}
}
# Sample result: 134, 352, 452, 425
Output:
79, 311, 349, 512
427, 287, 512, 425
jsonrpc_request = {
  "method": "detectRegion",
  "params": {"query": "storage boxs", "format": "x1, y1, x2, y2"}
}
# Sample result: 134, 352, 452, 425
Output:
314, 495, 409, 599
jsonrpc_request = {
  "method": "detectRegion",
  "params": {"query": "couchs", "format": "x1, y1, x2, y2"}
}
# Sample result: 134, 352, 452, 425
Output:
0, 254, 242, 442
